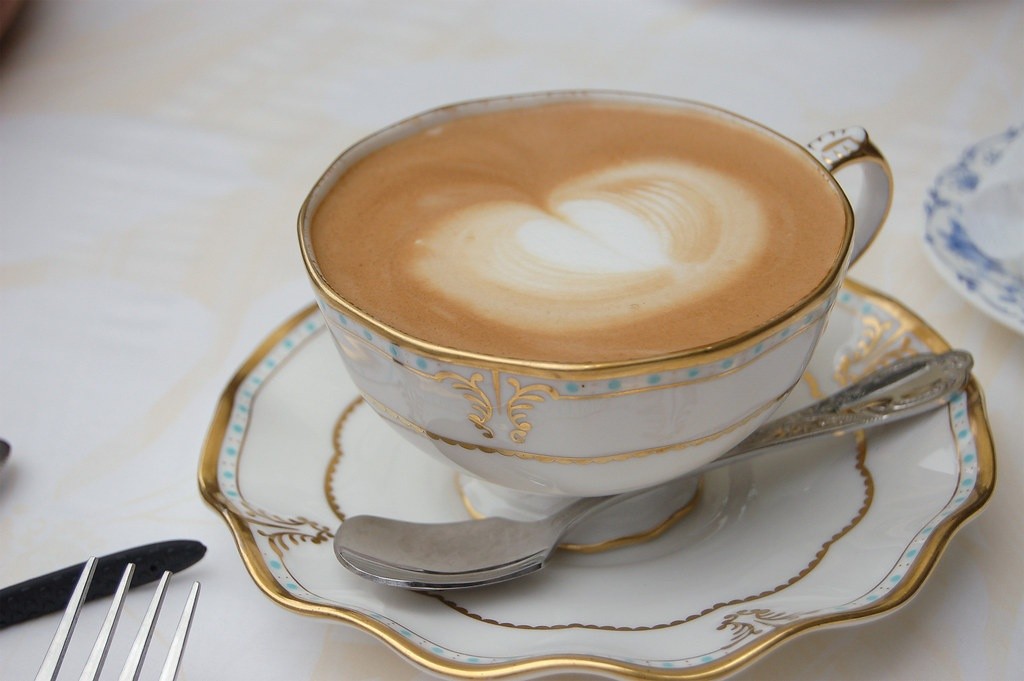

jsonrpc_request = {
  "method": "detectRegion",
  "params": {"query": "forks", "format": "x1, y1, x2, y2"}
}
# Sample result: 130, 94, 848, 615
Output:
32, 556, 217, 681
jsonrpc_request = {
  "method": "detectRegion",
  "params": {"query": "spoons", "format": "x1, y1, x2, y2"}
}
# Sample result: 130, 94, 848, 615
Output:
334, 347, 974, 593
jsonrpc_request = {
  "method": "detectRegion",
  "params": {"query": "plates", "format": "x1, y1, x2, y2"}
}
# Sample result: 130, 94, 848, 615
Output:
195, 273, 999, 681
919, 119, 1024, 337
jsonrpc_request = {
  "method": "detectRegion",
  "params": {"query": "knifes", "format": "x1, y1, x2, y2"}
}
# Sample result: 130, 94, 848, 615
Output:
0, 539, 207, 632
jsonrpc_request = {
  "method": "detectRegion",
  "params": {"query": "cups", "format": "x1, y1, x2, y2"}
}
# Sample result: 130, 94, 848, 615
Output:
299, 88, 896, 502
